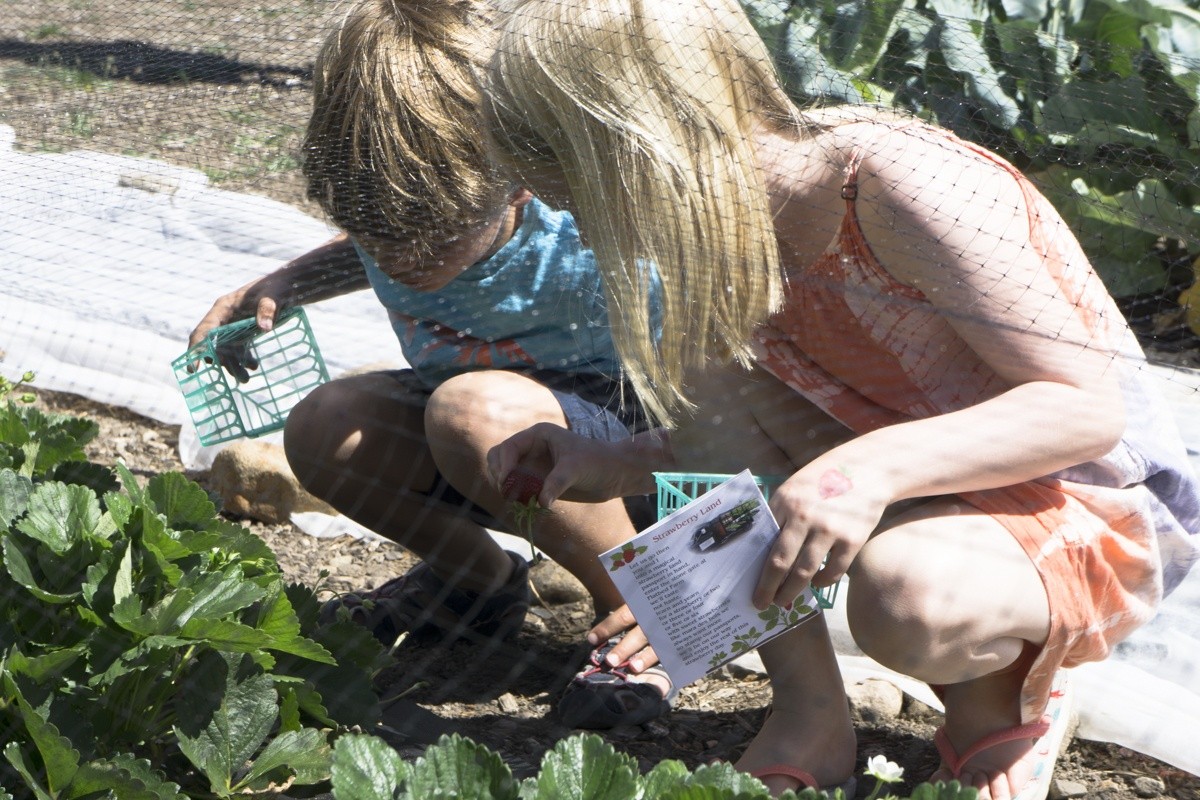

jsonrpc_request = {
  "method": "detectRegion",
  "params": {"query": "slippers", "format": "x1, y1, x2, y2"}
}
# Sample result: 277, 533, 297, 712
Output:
745, 766, 857, 800
932, 670, 1080, 800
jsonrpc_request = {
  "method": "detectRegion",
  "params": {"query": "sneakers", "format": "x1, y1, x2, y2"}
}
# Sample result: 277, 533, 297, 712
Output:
556, 632, 679, 725
317, 550, 528, 641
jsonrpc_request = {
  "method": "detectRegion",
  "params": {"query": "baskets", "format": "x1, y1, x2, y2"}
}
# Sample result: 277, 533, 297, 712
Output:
172, 306, 330, 445
653, 468, 839, 619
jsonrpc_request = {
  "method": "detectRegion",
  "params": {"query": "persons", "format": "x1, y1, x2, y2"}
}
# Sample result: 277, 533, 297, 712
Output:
184, 0, 675, 728
482, 0, 1200, 800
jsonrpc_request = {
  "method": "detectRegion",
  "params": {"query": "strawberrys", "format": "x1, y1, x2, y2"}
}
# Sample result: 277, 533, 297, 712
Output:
502, 469, 543, 506
